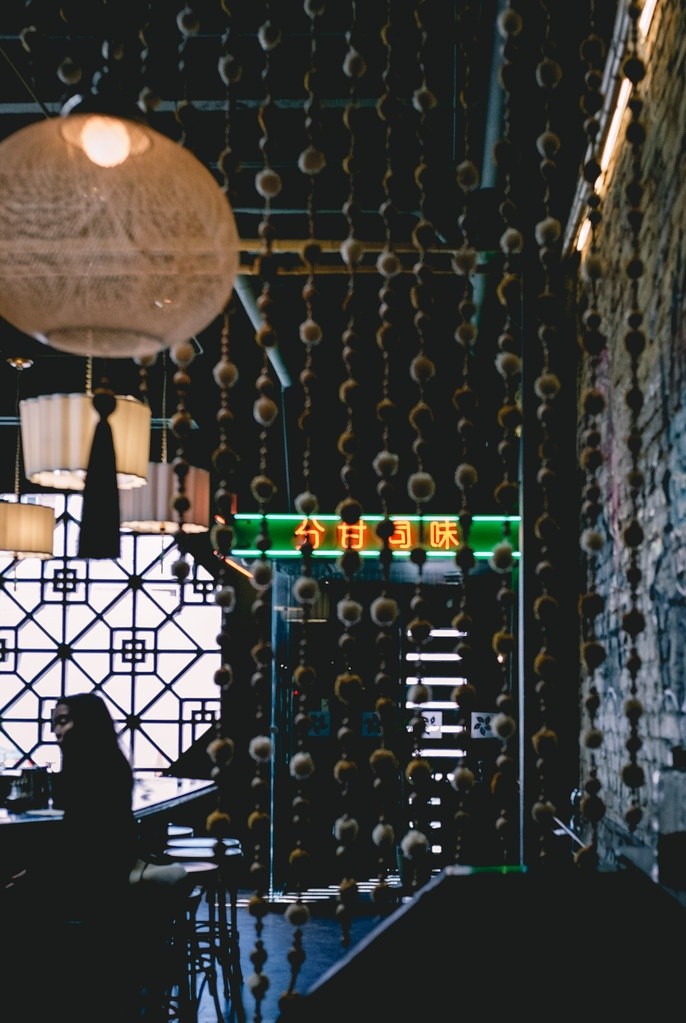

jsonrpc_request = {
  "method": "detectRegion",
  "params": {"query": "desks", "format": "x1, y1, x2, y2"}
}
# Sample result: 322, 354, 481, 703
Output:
0, 808, 69, 878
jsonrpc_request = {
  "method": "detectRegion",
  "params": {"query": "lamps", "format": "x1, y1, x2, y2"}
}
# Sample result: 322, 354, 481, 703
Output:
0, 115, 240, 559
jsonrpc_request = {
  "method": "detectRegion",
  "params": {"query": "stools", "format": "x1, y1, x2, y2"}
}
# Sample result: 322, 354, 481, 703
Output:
157, 825, 246, 1023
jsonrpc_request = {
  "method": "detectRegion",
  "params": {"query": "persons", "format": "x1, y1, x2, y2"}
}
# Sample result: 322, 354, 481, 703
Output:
0, 694, 136, 920
